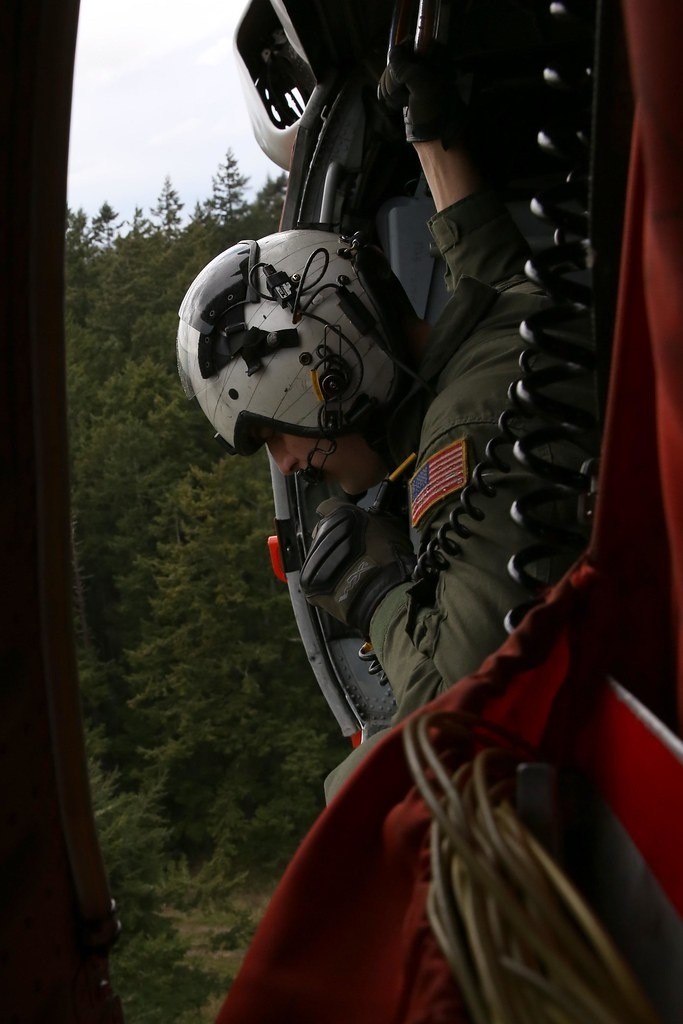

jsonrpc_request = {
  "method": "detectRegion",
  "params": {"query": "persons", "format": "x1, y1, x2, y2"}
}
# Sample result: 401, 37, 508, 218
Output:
171, 41, 614, 726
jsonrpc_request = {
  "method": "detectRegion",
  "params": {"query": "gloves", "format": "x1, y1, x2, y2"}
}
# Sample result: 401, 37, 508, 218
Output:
296, 496, 410, 631
375, 37, 472, 151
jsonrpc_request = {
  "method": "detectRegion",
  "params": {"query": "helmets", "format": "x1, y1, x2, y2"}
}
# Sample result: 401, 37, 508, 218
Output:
176, 230, 411, 487
